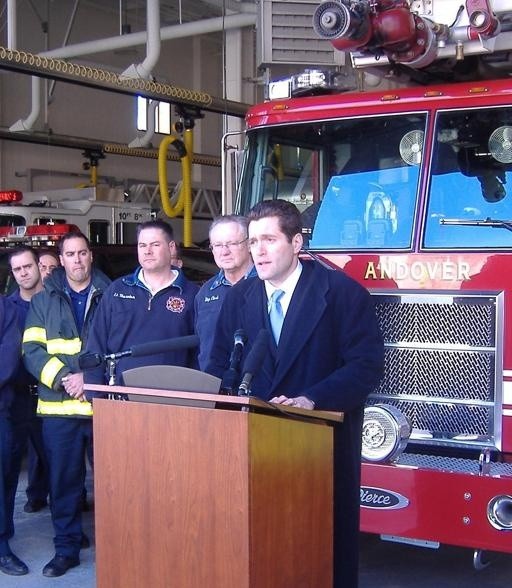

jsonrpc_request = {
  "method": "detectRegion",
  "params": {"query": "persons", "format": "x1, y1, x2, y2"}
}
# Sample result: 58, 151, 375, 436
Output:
0, 292, 30, 576
204, 199, 384, 588
5, 244, 90, 550
82, 220, 201, 407
21, 232, 112, 578
193, 215, 258, 373
24, 249, 89, 512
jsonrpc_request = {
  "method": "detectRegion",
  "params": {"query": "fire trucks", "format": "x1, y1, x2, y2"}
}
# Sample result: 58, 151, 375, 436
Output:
0, 186, 153, 247
219, 2, 512, 569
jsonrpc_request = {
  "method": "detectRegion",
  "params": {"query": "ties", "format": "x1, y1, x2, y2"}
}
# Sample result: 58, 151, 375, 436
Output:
269, 289, 284, 346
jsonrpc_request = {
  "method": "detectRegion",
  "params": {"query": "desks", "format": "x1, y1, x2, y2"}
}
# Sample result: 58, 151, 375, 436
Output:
83, 383, 343, 588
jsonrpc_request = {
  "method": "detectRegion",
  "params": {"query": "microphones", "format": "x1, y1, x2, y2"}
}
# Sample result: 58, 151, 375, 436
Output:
238, 330, 272, 396
230, 328, 248, 371
102, 335, 201, 358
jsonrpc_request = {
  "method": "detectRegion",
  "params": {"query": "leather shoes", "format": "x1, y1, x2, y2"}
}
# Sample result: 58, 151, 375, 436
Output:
43, 552, 80, 576
24, 496, 47, 512
0, 554, 28, 575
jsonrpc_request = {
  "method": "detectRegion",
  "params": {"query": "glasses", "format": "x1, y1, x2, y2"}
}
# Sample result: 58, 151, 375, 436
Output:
208, 237, 247, 249
